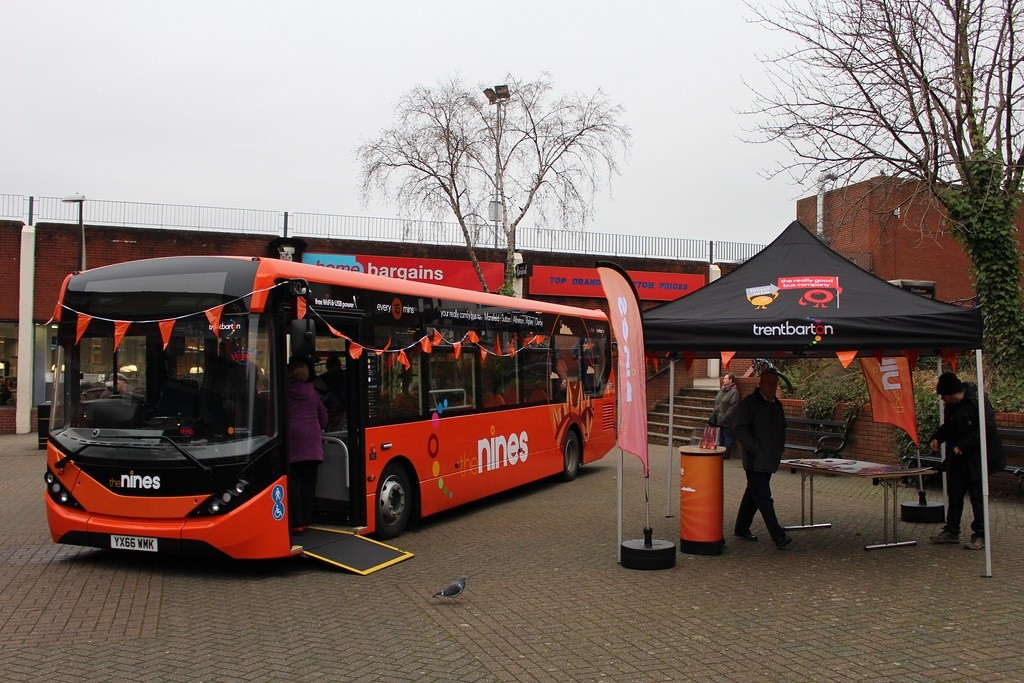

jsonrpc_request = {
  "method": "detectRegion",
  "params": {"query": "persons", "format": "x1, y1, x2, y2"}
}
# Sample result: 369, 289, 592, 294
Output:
286, 360, 330, 546
713, 372, 740, 460
322, 356, 347, 388
100, 372, 129, 399
730, 368, 794, 549
398, 382, 420, 412
929, 371, 1008, 550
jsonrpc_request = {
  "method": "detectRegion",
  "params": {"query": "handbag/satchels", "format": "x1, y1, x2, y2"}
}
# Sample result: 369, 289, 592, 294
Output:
950, 447, 981, 481
707, 410, 717, 427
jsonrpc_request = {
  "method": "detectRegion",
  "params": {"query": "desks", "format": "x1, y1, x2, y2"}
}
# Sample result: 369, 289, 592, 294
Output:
779, 458, 937, 550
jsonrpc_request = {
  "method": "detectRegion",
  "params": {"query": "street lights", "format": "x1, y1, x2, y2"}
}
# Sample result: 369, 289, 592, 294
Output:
483, 84, 510, 248
58, 193, 87, 270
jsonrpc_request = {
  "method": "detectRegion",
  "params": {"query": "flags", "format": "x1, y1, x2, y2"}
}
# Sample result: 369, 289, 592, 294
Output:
858, 357, 920, 451
596, 260, 650, 477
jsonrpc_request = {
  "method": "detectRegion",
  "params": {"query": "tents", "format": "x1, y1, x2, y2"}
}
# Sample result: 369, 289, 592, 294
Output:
617, 219, 992, 577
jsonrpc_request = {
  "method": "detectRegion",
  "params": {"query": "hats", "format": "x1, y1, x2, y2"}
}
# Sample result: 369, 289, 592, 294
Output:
108, 372, 133, 380
936, 371, 963, 395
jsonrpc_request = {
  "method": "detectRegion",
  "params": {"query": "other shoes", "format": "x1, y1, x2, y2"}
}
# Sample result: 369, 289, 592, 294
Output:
304, 524, 309, 530
775, 534, 792, 549
291, 526, 304, 536
734, 532, 758, 541
723, 455, 730, 460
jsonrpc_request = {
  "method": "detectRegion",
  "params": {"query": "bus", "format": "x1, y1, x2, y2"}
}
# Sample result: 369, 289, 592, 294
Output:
41, 250, 623, 567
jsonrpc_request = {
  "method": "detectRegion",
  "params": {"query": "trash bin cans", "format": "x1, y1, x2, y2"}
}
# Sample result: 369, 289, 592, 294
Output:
37, 402, 51, 449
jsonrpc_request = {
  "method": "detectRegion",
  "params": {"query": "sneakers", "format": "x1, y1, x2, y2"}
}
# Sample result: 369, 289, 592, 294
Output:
964, 536, 985, 550
929, 530, 960, 543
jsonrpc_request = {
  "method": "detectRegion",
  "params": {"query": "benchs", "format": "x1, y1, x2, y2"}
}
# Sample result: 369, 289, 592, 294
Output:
785, 414, 854, 476
903, 427, 1024, 487
